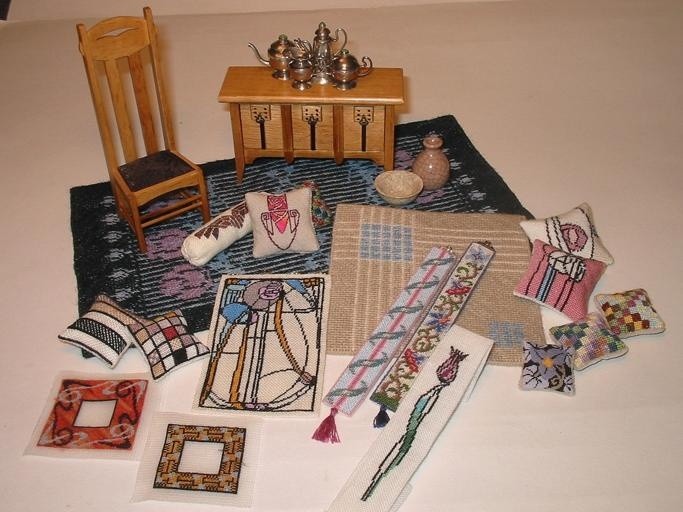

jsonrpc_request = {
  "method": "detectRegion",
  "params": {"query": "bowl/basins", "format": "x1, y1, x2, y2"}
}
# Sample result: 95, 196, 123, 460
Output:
374, 170, 424, 206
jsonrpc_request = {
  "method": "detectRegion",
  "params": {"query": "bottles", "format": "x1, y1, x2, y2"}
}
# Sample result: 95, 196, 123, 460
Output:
411, 137, 450, 191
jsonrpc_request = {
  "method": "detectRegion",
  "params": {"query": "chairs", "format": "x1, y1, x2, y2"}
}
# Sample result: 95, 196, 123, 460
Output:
75, 6, 213, 253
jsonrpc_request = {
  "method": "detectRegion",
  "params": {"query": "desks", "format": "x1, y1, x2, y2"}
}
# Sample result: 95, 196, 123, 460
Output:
218, 67, 407, 186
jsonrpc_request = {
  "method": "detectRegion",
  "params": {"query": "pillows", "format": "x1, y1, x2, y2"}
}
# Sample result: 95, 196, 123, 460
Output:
57, 291, 211, 385
514, 197, 665, 400
180, 181, 333, 267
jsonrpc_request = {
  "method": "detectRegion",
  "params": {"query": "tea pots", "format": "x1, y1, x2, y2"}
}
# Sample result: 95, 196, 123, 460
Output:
247, 21, 373, 92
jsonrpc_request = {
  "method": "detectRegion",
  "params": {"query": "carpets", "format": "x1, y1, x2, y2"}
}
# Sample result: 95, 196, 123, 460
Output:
70, 113, 536, 359
